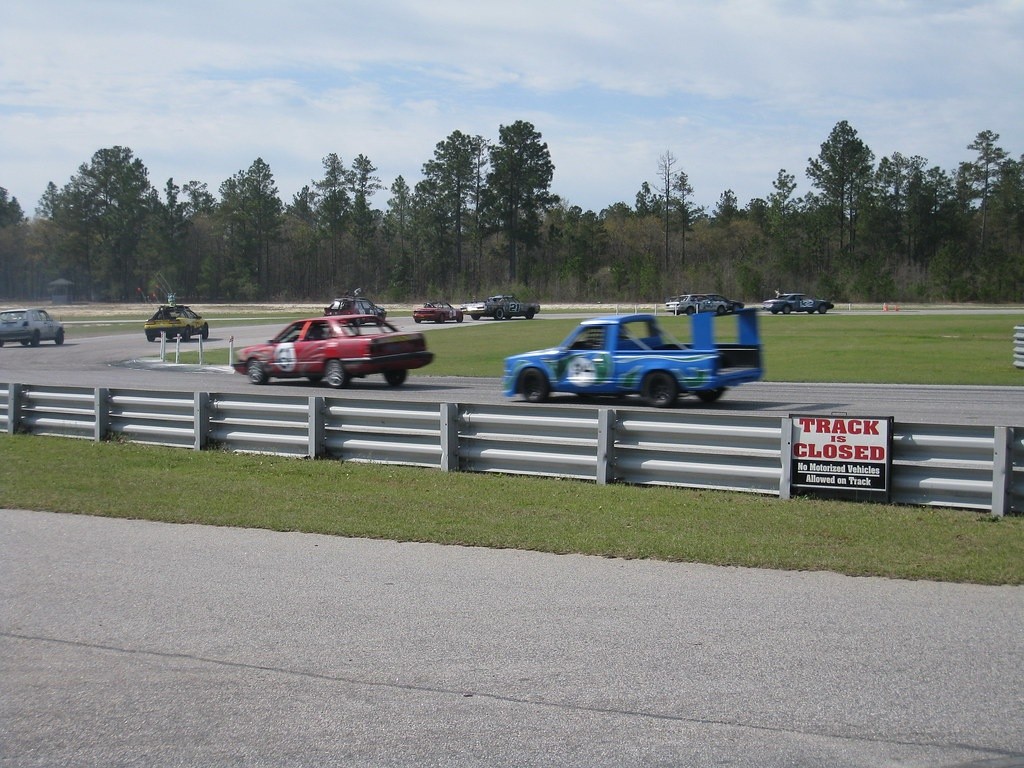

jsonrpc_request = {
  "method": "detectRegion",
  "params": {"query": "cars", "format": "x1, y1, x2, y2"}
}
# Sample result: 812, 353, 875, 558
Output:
461, 296, 541, 320
413, 301, 463, 324
698, 294, 745, 314
763, 294, 834, 314
666, 294, 728, 316
144, 294, 209, 342
322, 288, 387, 326
231, 315, 435, 388
0, 310, 65, 348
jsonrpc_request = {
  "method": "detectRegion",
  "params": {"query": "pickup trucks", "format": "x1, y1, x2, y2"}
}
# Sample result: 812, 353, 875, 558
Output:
502, 307, 764, 408
